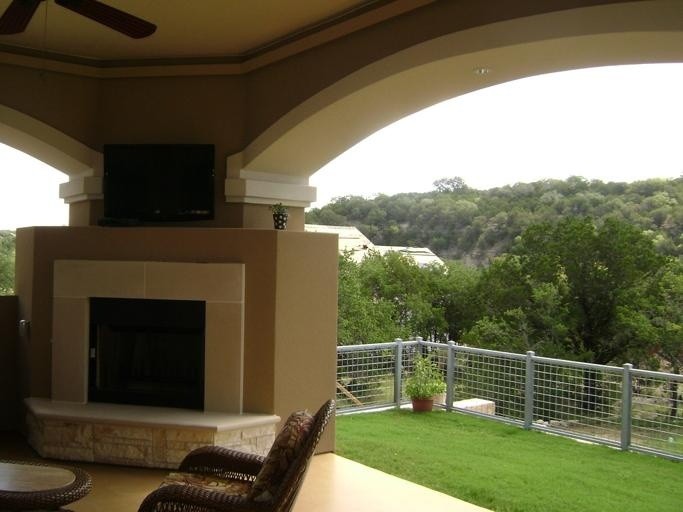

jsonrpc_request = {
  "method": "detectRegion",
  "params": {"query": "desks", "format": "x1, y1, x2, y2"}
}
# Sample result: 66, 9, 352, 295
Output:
0, 459, 92, 512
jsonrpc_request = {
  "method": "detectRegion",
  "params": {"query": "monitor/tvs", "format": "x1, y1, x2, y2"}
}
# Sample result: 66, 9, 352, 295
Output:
103, 143, 215, 222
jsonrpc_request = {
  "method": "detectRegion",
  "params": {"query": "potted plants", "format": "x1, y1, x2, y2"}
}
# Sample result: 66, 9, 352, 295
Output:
405, 353, 447, 412
270, 203, 289, 229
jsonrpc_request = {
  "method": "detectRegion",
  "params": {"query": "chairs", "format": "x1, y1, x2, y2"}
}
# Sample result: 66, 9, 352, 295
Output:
138, 399, 335, 512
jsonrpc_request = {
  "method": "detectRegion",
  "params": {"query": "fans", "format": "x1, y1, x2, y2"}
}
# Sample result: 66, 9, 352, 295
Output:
0, 0, 159, 39
